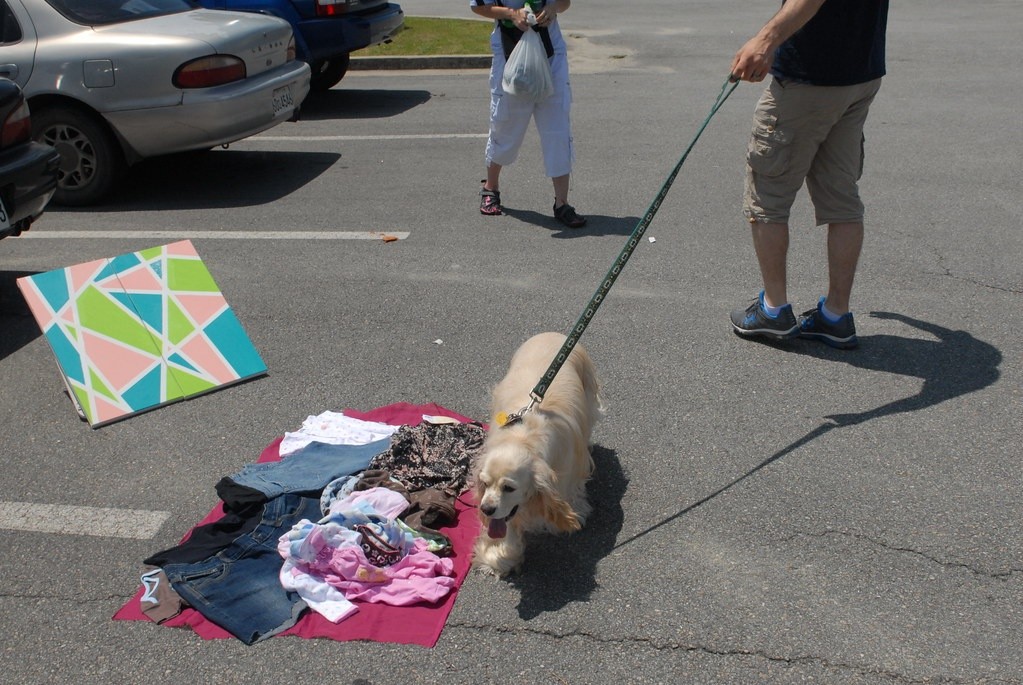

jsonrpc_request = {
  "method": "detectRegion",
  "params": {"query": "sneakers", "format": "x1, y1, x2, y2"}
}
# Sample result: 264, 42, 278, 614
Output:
552, 197, 586, 227
728, 288, 801, 340
796, 297, 857, 346
480, 179, 501, 215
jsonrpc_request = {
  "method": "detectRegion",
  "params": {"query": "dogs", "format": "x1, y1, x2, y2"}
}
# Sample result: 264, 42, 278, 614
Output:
474, 332, 606, 572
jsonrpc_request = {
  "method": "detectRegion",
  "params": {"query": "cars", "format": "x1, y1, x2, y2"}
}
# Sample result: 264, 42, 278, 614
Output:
0, 0, 406, 240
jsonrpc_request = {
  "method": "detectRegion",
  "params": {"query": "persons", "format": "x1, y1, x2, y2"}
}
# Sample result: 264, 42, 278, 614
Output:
468, 0, 587, 228
728, 1, 890, 349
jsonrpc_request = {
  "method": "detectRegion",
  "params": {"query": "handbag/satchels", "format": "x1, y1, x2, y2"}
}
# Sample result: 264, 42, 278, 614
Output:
502, 13, 554, 100
